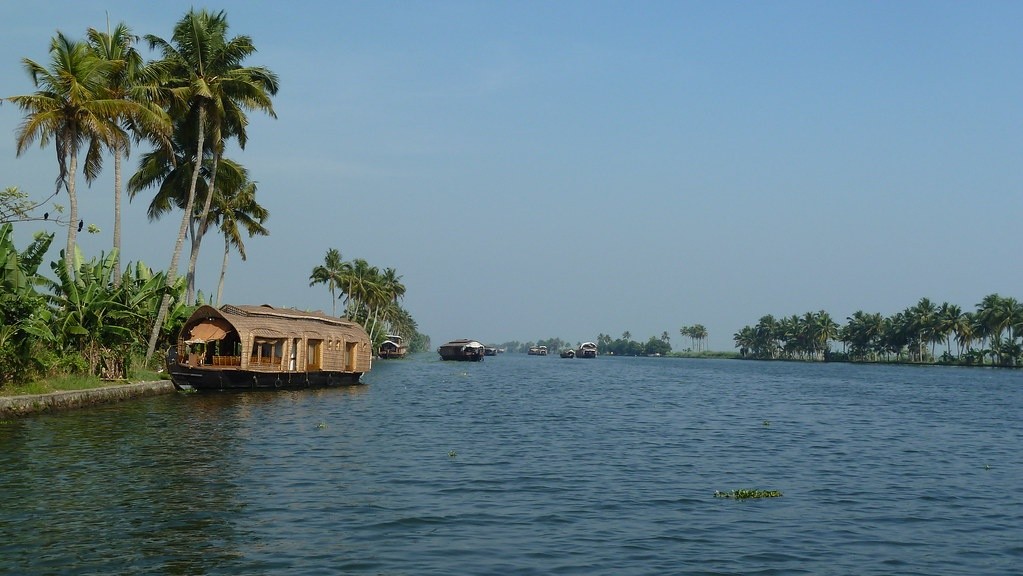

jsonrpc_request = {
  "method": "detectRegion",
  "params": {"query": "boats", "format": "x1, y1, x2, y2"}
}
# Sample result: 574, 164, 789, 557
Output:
164, 303, 371, 391
497, 348, 504, 354
377, 334, 409, 359
560, 348, 575, 358
485, 346, 497, 356
537, 346, 547, 356
527, 346, 538, 355
576, 342, 598, 358
648, 352, 661, 358
437, 338, 485, 362
